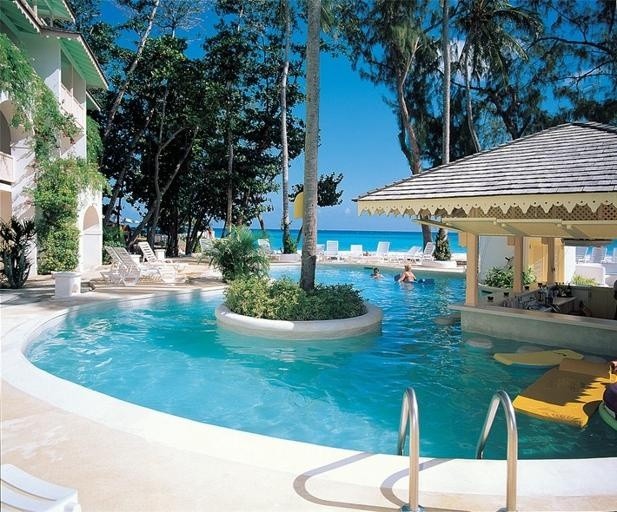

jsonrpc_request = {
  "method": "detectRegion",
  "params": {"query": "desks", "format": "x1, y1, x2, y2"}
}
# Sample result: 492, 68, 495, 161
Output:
536, 296, 577, 314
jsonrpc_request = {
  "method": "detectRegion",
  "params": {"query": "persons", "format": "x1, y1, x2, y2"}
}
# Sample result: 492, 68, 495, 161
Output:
397, 263, 416, 284
370, 268, 385, 280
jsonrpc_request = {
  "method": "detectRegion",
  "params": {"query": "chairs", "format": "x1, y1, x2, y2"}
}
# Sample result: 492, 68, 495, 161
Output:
575, 246, 617, 275
100, 237, 190, 286
0, 463, 81, 512
196, 237, 439, 269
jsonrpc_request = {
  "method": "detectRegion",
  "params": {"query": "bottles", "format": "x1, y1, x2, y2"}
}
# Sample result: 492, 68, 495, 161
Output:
536, 282, 572, 307
